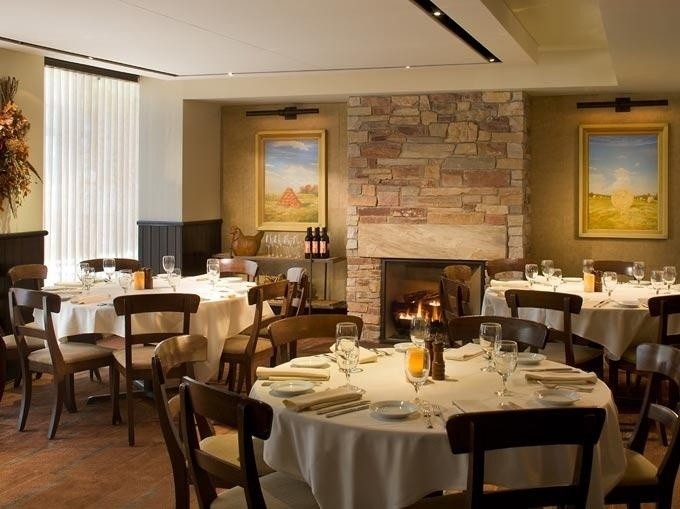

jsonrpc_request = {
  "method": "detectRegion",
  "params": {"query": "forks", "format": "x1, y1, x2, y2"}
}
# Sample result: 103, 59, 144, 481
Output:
536, 379, 593, 391
370, 348, 392, 358
430, 398, 447, 429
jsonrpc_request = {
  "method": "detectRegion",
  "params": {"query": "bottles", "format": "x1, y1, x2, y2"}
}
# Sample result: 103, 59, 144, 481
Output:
304, 226, 330, 259
431, 342, 447, 380
424, 338, 436, 376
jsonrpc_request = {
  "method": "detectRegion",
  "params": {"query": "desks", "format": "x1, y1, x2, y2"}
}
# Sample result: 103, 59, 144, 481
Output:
479, 269, 680, 403
34, 273, 278, 426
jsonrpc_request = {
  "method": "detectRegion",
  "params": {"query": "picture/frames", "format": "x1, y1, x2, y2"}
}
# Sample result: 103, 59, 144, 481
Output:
253, 127, 330, 236
576, 120, 672, 241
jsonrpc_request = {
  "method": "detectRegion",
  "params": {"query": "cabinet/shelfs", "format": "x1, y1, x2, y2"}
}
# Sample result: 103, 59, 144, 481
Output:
207, 250, 347, 310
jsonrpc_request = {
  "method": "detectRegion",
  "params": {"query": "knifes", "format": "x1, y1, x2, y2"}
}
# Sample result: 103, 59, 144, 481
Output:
326, 405, 369, 418
316, 400, 369, 416
420, 397, 432, 431
520, 365, 576, 373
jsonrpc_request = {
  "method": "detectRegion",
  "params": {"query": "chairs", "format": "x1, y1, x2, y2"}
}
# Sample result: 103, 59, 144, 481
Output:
207, 255, 259, 280
223, 267, 308, 385
216, 279, 292, 392
421, 400, 608, 508
177, 371, 329, 509
109, 293, 202, 445
6, 286, 124, 445
80, 258, 143, 281
265, 312, 365, 367
608, 338, 680, 508
7, 262, 50, 314
441, 258, 680, 447
3, 313, 46, 393
148, 333, 278, 509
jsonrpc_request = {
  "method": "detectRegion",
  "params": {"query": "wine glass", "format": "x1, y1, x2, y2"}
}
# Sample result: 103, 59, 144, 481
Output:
404, 347, 432, 411
488, 258, 677, 307
337, 337, 366, 395
263, 231, 305, 259
490, 339, 518, 396
41, 255, 221, 300
480, 321, 504, 372
409, 318, 427, 349
335, 322, 360, 373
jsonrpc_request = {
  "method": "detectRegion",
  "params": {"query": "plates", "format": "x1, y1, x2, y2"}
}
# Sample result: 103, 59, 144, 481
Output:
533, 386, 581, 406
291, 356, 329, 369
394, 343, 412, 354
507, 348, 546, 366
370, 400, 419, 418
270, 379, 314, 396
